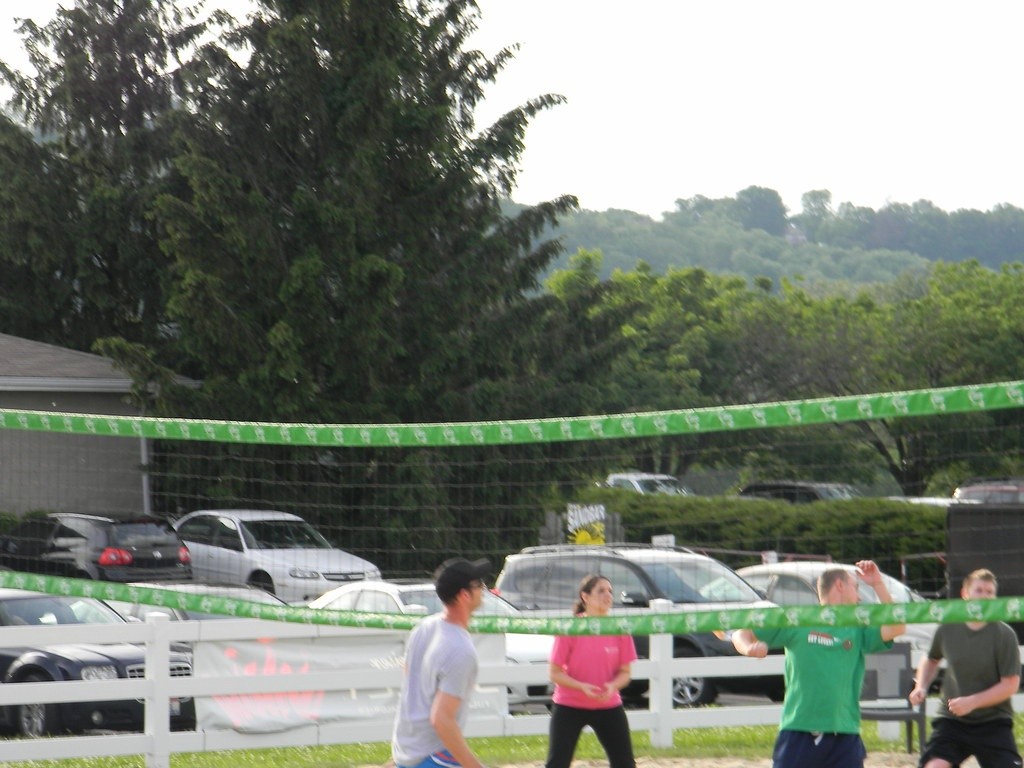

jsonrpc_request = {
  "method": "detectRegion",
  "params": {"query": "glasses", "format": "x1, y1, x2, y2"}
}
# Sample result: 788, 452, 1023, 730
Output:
468, 577, 485, 588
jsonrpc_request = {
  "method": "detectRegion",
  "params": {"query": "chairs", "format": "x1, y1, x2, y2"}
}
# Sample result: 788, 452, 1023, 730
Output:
860, 642, 926, 757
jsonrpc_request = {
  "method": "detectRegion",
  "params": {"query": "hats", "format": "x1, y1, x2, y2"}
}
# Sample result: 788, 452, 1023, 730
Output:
433, 556, 491, 593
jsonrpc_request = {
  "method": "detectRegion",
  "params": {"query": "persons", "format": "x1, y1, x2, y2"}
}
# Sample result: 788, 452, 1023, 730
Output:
544, 574, 637, 768
390, 558, 492, 768
908, 569, 1024, 768
731, 560, 906, 768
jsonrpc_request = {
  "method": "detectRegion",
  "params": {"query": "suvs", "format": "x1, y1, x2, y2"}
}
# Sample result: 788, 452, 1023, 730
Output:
489, 541, 797, 706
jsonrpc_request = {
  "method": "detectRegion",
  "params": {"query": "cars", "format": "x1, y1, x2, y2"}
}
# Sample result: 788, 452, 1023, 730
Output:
171, 508, 383, 603
740, 481, 864, 503
1, 511, 194, 584
0, 585, 293, 740
307, 581, 562, 705
600, 472, 693, 497
955, 481, 1023, 503
701, 558, 949, 688
